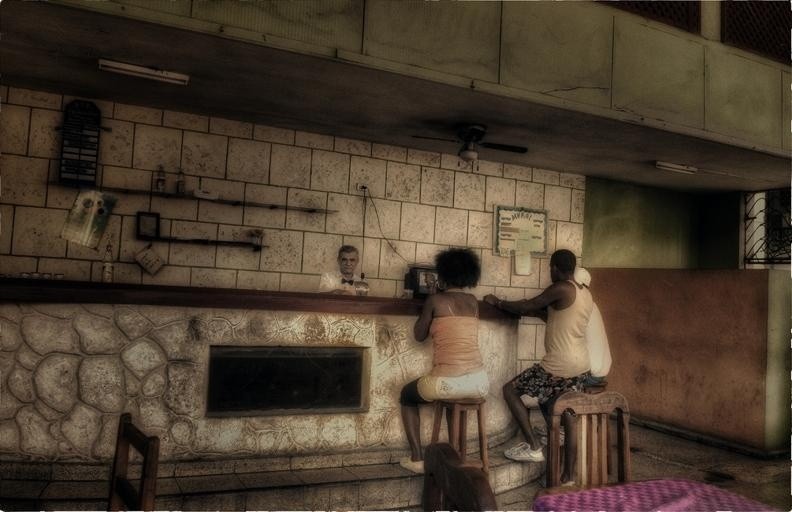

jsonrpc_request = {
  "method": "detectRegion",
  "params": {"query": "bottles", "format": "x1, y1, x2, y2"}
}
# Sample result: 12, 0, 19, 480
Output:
354, 273, 369, 296
175, 171, 185, 194
102, 244, 114, 282
155, 165, 167, 192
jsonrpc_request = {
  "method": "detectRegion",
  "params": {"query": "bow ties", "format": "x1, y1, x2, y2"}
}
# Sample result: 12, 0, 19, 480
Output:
340, 278, 354, 286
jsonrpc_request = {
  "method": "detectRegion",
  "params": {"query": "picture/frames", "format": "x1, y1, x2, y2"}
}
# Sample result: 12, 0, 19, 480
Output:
135, 209, 160, 238
492, 204, 550, 259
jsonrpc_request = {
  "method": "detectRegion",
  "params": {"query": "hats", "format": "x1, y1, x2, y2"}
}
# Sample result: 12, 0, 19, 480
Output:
573, 265, 592, 288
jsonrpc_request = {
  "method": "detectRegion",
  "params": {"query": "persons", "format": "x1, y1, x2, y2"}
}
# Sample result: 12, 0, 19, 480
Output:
397, 245, 492, 477
569, 263, 615, 389
482, 249, 595, 489
419, 269, 438, 294
319, 243, 372, 297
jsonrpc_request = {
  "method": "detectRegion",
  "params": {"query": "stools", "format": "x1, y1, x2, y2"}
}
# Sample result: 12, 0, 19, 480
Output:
430, 399, 489, 475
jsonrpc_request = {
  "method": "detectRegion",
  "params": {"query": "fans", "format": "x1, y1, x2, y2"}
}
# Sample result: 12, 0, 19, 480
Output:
409, 123, 528, 156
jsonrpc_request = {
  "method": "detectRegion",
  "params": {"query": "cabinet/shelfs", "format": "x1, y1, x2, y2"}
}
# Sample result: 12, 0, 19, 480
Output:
45, 177, 340, 252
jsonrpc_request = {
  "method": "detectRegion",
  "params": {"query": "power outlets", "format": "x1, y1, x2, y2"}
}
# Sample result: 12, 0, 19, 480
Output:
356, 183, 367, 192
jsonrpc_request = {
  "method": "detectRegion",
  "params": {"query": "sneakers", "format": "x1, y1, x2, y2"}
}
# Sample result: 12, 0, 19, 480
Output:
503, 441, 546, 463
534, 425, 566, 447
398, 455, 426, 475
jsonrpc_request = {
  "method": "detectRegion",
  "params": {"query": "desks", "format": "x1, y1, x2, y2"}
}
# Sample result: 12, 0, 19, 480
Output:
535, 476, 783, 511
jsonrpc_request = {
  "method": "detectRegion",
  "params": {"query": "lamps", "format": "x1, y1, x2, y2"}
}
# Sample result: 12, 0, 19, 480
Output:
654, 159, 698, 175
97, 58, 190, 86
458, 142, 483, 163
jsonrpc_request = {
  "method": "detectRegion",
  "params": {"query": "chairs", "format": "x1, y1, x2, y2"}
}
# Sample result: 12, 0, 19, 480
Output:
545, 388, 629, 488
422, 441, 498, 511
106, 412, 159, 512
